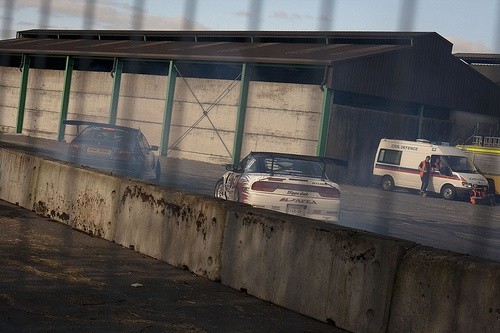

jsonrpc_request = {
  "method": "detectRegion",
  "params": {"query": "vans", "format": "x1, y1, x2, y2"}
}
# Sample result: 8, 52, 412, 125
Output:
374, 138, 492, 204
455, 145, 500, 199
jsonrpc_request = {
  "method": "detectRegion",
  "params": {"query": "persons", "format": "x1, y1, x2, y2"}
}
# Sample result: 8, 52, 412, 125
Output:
430, 158, 445, 168
469, 185, 487, 205
480, 186, 496, 206
418, 156, 431, 197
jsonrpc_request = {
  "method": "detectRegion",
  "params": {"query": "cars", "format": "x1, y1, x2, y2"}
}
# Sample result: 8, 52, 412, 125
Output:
214, 151, 342, 224
66, 124, 162, 184
